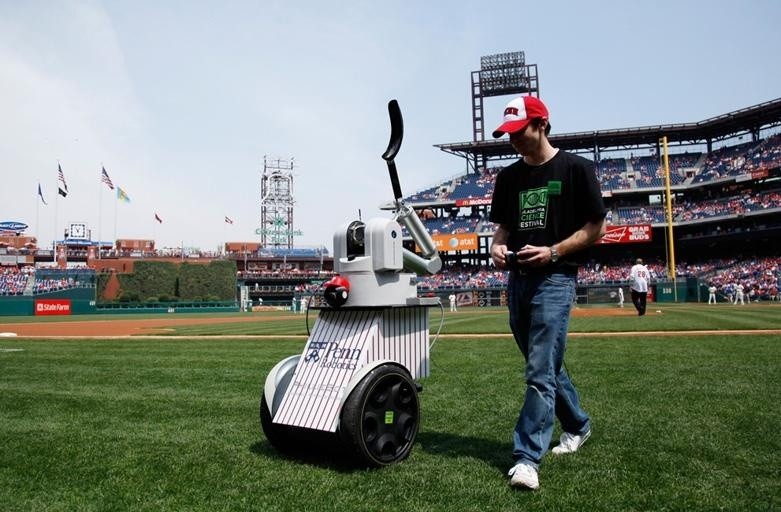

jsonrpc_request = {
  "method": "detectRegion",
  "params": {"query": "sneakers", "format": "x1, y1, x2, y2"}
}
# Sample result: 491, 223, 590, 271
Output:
552, 427, 592, 454
508, 462, 540, 490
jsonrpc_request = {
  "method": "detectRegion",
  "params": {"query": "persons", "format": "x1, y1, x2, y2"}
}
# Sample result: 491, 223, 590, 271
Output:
1, 265, 97, 297
629, 258, 649, 317
300, 296, 307, 314
291, 296, 296, 313
480, 96, 608, 489
448, 291, 457, 312
386, 131, 781, 308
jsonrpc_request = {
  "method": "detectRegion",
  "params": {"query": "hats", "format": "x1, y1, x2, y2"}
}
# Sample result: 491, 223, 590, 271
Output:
493, 95, 550, 138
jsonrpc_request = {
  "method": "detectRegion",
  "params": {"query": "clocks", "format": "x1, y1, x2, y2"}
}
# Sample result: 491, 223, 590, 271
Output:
71, 224, 86, 239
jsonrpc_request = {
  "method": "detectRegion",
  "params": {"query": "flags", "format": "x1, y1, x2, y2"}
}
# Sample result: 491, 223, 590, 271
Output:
155, 213, 163, 223
117, 185, 130, 203
101, 167, 114, 190
57, 162, 69, 198
38, 181, 48, 208
225, 215, 232, 225
